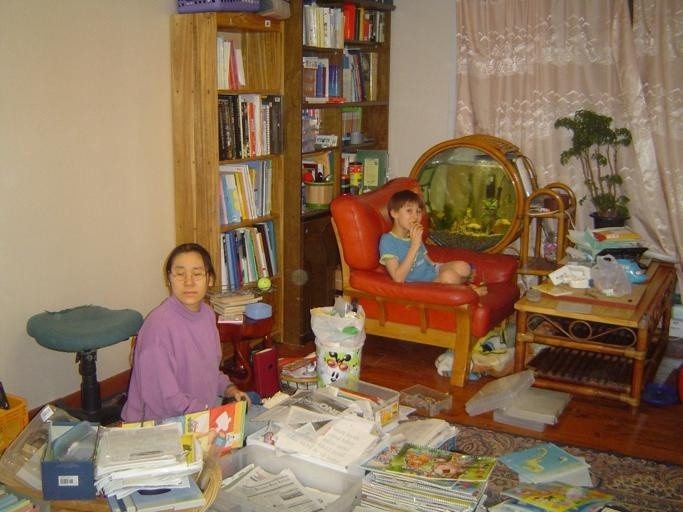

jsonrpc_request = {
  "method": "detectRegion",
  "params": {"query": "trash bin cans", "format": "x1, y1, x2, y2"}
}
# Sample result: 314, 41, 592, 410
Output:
314, 340, 365, 389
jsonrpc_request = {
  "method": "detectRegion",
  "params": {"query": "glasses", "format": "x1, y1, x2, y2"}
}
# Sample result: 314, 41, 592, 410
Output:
168, 270, 209, 281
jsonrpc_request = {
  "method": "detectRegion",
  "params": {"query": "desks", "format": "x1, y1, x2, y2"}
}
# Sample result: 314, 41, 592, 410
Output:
210, 315, 272, 391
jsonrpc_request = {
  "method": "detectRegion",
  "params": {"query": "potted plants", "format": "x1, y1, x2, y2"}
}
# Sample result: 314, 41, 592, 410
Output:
554, 110, 631, 228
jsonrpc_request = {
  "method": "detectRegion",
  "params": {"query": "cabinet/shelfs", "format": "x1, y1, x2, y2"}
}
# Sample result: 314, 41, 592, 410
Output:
286, 1, 389, 347
171, 12, 286, 345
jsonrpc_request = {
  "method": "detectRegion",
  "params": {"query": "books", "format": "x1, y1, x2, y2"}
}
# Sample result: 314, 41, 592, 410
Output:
210, 37, 277, 324
303, 2, 389, 195
0, 354, 623, 512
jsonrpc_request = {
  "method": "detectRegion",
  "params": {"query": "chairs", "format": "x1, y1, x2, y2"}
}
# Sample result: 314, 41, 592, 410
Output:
330, 175, 521, 389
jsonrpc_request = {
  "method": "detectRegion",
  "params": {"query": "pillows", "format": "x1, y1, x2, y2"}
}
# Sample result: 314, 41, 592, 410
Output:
330, 177, 430, 271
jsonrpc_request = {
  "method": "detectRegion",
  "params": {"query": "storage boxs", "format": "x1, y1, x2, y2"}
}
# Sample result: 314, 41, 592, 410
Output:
1, 377, 400, 512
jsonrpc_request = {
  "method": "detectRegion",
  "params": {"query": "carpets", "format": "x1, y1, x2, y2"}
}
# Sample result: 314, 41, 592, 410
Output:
397, 413, 683, 512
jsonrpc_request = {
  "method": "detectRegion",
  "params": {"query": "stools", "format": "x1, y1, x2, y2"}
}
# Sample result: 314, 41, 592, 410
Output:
26, 305, 145, 426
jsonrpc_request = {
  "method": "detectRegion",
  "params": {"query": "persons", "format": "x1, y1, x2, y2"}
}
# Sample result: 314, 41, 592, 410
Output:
374, 187, 490, 300
114, 239, 278, 446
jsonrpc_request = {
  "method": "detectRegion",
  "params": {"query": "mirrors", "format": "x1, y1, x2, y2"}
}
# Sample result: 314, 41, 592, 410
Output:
409, 134, 539, 254
